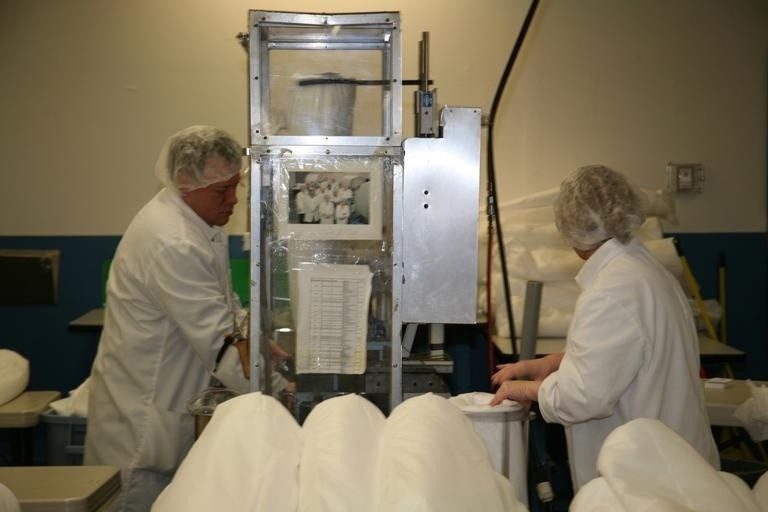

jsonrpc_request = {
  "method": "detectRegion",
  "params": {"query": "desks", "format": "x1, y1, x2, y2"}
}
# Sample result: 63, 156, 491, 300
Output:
1, 308, 122, 511
489, 330, 745, 360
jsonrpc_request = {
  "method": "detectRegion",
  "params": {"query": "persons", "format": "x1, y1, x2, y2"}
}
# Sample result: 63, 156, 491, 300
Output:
294, 173, 370, 225
489, 164, 722, 489
84, 124, 293, 512
